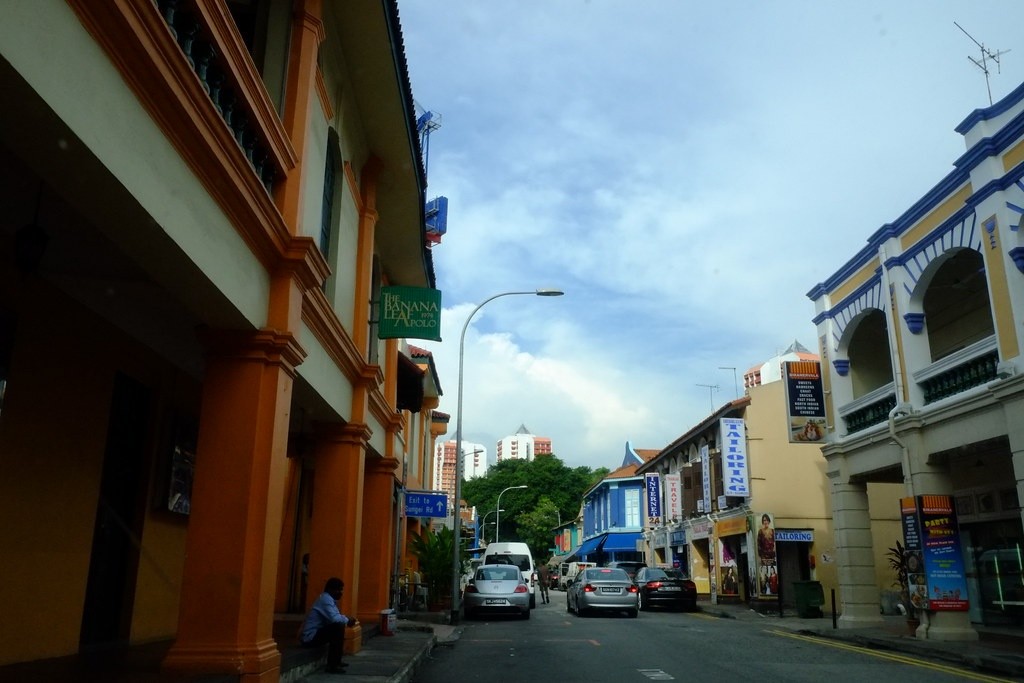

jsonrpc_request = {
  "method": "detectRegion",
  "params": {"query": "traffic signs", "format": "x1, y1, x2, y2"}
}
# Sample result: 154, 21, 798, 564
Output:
406, 493, 447, 517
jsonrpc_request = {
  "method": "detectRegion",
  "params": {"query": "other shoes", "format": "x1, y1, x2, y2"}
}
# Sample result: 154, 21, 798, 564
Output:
338, 662, 349, 667
546, 597, 549, 603
326, 667, 345, 674
542, 602, 545, 604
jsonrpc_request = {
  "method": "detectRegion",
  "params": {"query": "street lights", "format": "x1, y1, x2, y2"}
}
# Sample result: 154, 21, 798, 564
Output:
451, 289, 565, 626
496, 485, 528, 544
477, 509, 506, 547
554, 511, 561, 526
449, 450, 484, 529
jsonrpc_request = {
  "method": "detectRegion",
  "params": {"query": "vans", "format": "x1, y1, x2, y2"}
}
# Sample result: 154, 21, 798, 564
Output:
482, 542, 535, 609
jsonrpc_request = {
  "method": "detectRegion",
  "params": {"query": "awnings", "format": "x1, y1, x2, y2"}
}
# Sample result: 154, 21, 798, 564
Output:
576, 534, 606, 556
602, 533, 644, 552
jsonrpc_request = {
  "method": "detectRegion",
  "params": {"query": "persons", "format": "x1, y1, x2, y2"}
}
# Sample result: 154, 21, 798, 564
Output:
723, 568, 735, 594
766, 566, 777, 594
538, 560, 550, 604
302, 578, 356, 674
757, 515, 775, 566
302, 553, 309, 582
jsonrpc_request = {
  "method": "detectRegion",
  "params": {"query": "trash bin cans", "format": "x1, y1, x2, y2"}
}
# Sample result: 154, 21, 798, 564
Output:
382, 612, 397, 636
881, 590, 900, 615
791, 580, 825, 619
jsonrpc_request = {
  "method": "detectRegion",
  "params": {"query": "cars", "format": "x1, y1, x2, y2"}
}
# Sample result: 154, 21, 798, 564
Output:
633, 566, 697, 611
566, 567, 639, 618
547, 569, 559, 590
464, 564, 530, 619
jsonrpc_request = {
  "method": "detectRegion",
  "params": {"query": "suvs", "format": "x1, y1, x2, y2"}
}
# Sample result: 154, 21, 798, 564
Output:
605, 561, 647, 581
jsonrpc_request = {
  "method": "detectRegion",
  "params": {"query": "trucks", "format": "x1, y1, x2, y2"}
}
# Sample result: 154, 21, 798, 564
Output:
559, 562, 596, 591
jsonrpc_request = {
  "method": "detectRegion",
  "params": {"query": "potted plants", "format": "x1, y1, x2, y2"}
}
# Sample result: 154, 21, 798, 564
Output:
887, 540, 918, 637
441, 551, 451, 604
